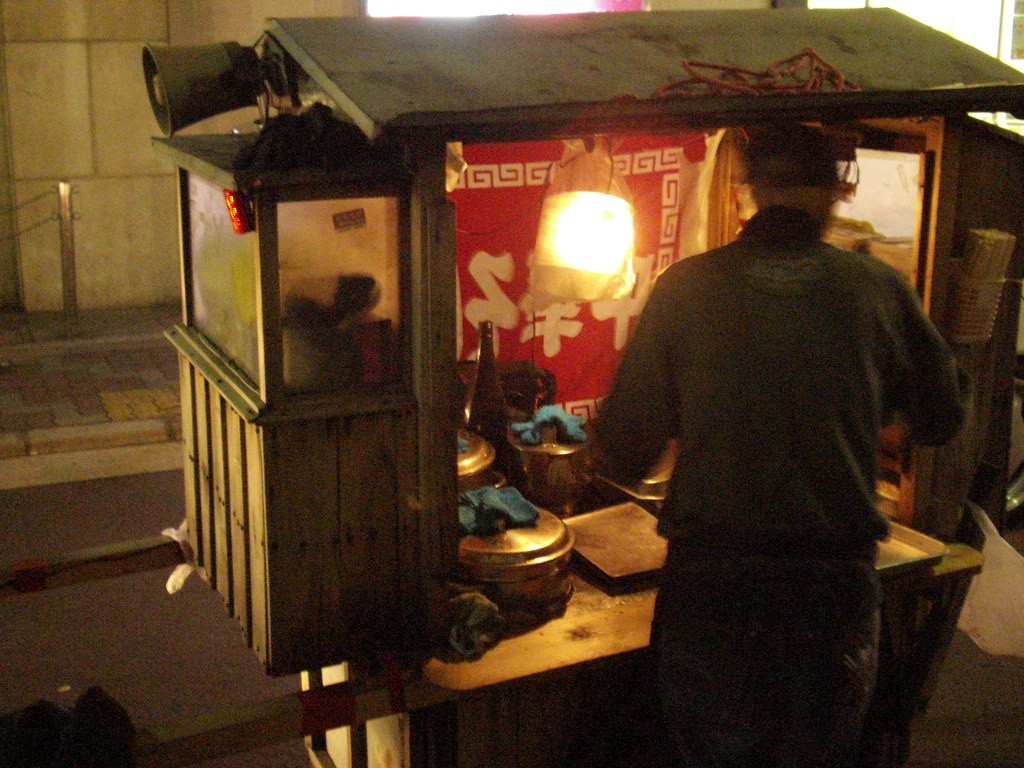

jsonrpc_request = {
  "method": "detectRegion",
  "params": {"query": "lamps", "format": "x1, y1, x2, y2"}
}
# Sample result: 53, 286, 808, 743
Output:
524, 144, 638, 309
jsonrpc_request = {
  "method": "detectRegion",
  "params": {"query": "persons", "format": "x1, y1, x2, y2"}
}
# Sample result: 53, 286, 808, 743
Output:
590, 119, 972, 768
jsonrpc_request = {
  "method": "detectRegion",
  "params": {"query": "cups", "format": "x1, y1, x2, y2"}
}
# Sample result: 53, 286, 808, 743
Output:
276, 270, 397, 398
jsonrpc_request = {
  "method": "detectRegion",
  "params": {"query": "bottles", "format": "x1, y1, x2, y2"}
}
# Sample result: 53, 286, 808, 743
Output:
471, 321, 507, 464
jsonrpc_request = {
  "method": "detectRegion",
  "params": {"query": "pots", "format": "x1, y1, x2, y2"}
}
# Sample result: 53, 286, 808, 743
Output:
455, 412, 681, 620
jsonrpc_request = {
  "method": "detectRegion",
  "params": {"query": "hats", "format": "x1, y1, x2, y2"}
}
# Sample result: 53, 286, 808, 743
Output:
747, 122, 840, 189
